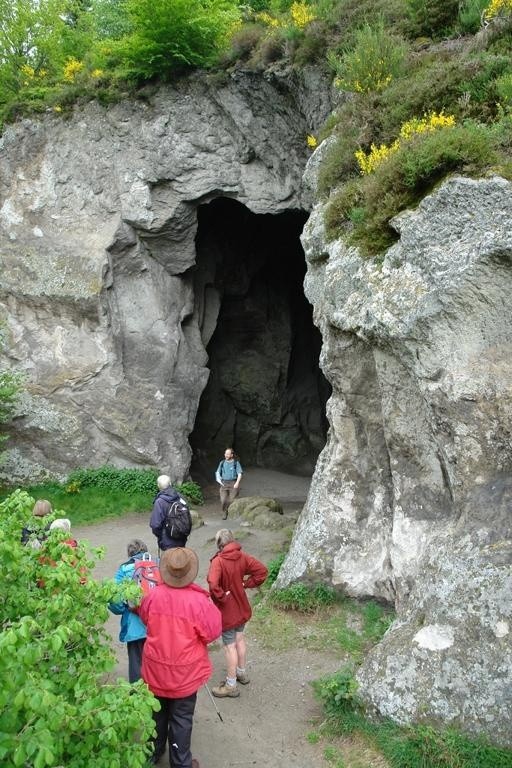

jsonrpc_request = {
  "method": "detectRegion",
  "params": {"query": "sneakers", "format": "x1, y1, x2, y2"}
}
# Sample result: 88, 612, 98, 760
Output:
213, 681, 240, 697
237, 673, 250, 684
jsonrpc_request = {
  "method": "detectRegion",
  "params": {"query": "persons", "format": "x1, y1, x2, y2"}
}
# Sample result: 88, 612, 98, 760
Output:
21, 501, 53, 561
215, 448, 243, 520
108, 538, 160, 696
138, 548, 223, 768
38, 518, 87, 589
207, 529, 268, 698
149, 475, 187, 559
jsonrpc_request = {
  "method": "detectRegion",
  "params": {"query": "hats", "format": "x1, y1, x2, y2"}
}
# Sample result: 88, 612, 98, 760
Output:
159, 547, 199, 588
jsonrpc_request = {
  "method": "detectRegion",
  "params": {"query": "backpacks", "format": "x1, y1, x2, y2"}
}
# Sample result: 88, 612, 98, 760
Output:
161, 497, 191, 539
127, 559, 162, 611
